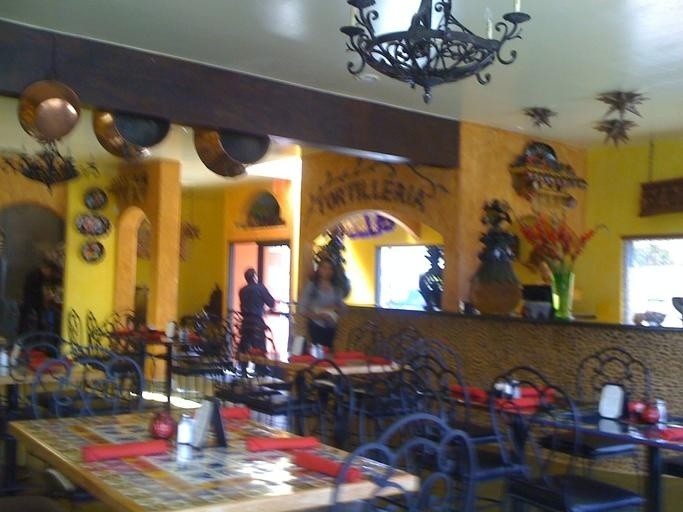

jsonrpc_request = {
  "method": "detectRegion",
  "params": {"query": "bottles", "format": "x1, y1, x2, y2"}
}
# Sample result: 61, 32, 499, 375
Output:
176, 413, 197, 444
165, 320, 177, 338
150, 410, 176, 440
640, 400, 668, 424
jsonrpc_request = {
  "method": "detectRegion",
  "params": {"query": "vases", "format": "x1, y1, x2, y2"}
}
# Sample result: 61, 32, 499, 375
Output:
546, 271, 574, 320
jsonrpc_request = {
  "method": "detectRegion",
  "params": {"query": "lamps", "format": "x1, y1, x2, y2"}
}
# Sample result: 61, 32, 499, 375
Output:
340, 0, 530, 104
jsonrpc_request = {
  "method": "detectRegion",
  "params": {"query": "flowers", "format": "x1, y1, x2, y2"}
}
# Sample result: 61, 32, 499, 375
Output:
519, 207, 608, 269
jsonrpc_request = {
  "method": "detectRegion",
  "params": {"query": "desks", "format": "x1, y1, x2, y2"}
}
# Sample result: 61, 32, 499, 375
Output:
7, 410, 421, 512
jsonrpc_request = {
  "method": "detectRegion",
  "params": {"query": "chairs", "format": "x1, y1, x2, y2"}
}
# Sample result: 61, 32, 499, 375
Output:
536, 345, 651, 494
10, 308, 155, 411
329, 412, 477, 511
1, 308, 104, 496
488, 365, 646, 511
107, 308, 456, 469
400, 340, 528, 510
30, 345, 143, 418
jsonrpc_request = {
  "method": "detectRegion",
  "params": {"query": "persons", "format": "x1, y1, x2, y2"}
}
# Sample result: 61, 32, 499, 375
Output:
238, 267, 276, 377
296, 256, 347, 354
